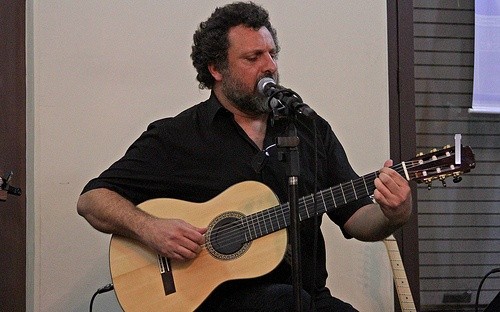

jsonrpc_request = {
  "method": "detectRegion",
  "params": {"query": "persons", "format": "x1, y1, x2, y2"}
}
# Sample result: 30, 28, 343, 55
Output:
76, 2, 412, 312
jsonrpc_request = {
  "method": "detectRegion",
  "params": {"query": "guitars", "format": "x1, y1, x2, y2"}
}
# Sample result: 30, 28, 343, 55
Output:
108, 135, 477, 311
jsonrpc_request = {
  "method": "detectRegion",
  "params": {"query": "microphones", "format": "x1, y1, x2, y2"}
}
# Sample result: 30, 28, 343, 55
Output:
257, 77, 313, 117
